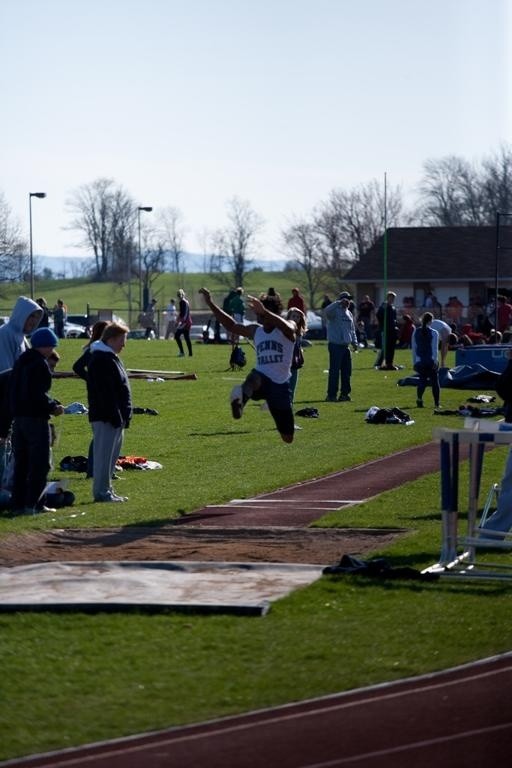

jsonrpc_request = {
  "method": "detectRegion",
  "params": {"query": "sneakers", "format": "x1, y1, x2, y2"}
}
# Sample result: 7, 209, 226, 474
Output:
338, 394, 355, 403
434, 408, 442, 412
325, 394, 339, 402
374, 364, 397, 372
111, 473, 125, 481
229, 384, 245, 421
93, 488, 129, 504
416, 399, 424, 408
0, 504, 57, 518
177, 352, 193, 358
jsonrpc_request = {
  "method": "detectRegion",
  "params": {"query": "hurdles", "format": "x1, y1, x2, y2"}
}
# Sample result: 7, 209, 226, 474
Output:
421, 419, 512, 582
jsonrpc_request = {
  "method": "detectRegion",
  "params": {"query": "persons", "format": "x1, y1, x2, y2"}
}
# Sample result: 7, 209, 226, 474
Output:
197, 287, 312, 443
322, 291, 512, 370
475, 349, 512, 541
138, 289, 193, 356
322, 292, 357, 402
1, 296, 68, 515
72, 320, 132, 502
411, 313, 440, 408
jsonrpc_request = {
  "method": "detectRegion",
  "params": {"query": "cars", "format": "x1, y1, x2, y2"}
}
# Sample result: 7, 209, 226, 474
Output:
181, 325, 214, 340
0, 314, 100, 340
219, 308, 323, 342
127, 329, 156, 341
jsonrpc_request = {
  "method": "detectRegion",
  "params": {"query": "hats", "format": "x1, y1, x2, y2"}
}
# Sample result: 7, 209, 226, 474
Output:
31, 326, 59, 348
336, 291, 356, 301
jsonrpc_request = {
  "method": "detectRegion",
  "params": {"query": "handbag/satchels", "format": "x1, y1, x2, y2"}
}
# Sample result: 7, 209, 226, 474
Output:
229, 346, 247, 368
291, 347, 305, 370
420, 358, 439, 378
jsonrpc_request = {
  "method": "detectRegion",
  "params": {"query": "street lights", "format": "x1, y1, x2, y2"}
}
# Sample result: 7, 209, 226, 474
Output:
136, 206, 152, 308
29, 190, 48, 303
